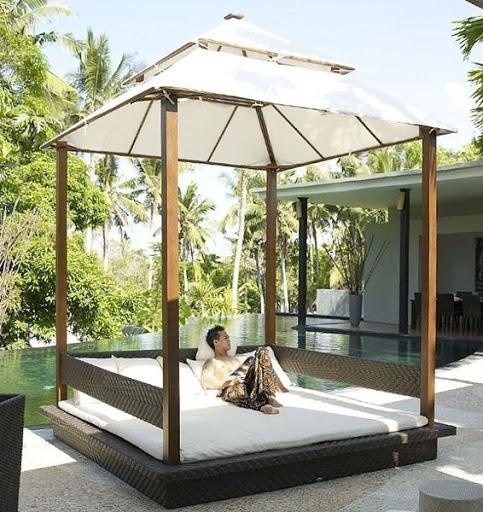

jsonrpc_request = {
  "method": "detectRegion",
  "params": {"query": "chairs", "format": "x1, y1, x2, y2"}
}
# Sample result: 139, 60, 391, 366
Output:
410, 290, 482, 336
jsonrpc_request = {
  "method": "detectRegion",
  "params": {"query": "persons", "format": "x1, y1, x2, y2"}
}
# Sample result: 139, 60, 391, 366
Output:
199, 323, 288, 417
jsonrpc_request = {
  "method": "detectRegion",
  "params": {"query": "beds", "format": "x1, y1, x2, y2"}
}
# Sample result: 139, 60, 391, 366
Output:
38, 11, 458, 509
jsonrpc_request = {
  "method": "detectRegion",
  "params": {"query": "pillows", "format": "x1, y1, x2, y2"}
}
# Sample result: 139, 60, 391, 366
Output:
68, 327, 291, 406
